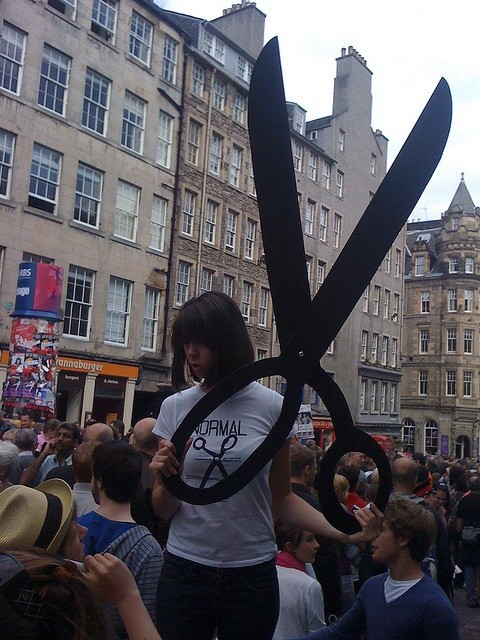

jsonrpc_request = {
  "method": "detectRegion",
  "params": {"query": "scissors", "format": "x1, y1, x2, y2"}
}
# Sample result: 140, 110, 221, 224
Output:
158, 36, 453, 534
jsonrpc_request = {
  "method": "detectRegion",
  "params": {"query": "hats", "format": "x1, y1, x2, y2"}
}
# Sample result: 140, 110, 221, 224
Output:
0, 479, 75, 556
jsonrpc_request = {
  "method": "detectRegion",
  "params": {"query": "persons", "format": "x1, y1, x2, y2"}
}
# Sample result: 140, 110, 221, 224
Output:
0, 414, 161, 640
149, 290, 385, 639
274, 436, 479, 640
